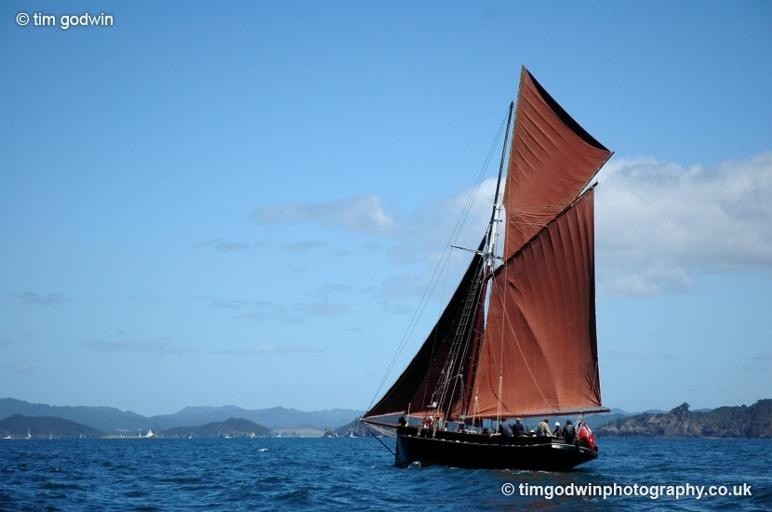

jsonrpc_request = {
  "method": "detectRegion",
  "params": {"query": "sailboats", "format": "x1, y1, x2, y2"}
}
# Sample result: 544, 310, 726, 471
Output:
348, 59, 617, 472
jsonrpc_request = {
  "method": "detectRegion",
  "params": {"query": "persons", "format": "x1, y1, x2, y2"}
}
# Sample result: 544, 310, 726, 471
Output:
561, 420, 575, 444
536, 418, 554, 438
551, 421, 562, 438
528, 429, 538, 438
498, 416, 514, 438
511, 417, 525, 438
